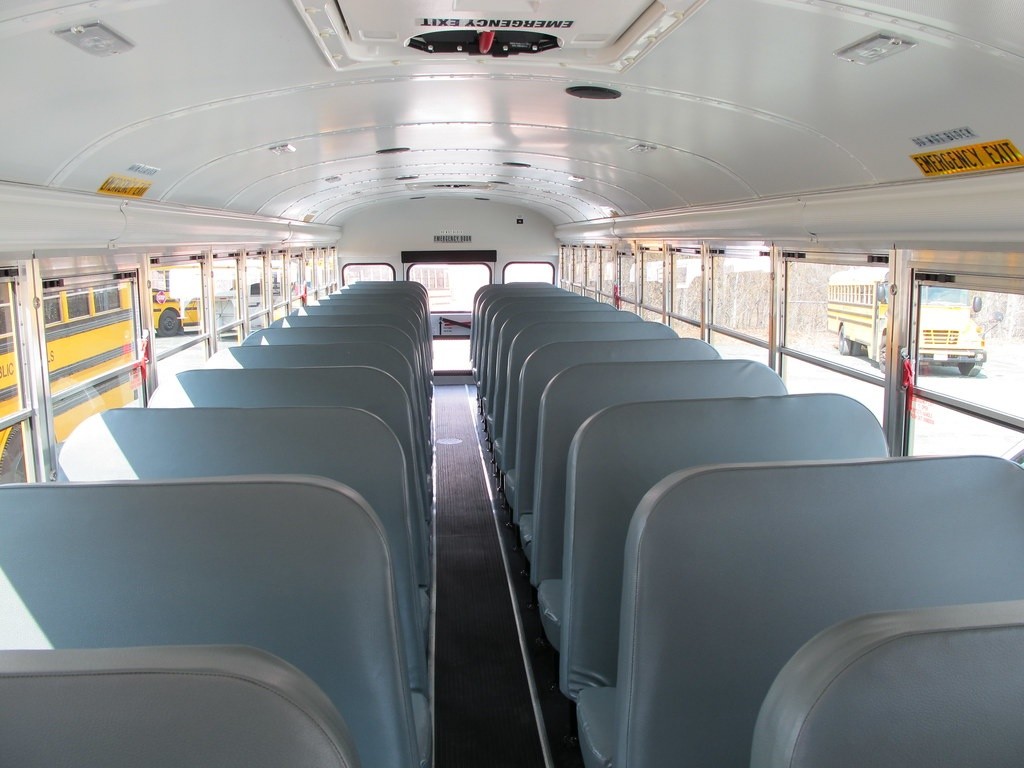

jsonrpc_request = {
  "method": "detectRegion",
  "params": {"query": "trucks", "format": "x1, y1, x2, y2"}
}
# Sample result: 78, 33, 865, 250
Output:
825, 267, 988, 376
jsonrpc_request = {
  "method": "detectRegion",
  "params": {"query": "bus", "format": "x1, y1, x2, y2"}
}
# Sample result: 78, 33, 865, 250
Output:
1, 248, 337, 486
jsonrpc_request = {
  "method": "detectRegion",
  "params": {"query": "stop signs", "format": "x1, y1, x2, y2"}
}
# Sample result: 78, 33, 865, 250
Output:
156, 291, 168, 304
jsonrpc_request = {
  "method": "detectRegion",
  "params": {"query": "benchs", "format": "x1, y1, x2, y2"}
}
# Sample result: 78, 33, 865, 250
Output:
471, 281, 1024, 768
1, 281, 436, 768
